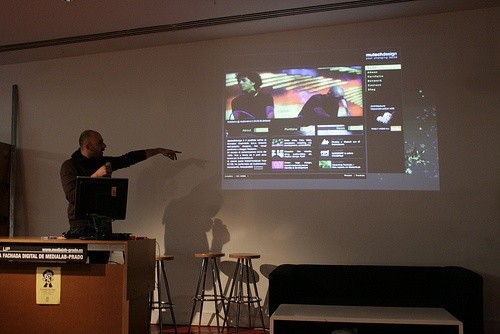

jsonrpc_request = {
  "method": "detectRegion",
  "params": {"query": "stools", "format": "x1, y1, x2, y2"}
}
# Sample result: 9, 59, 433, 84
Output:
187, 253, 229, 334
220, 252, 265, 334
150, 254, 177, 334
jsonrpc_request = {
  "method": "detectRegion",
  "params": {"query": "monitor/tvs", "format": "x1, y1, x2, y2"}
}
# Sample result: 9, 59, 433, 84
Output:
73, 176, 129, 241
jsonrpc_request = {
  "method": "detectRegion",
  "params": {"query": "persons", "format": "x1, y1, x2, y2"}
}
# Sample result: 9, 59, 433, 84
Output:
297, 85, 345, 116
59, 130, 182, 238
230, 70, 276, 119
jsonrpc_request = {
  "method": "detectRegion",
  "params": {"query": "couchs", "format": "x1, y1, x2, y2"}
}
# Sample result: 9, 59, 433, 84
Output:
267, 263, 483, 334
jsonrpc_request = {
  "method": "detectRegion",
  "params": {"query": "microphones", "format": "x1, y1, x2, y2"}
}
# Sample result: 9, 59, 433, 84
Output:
105, 162, 111, 166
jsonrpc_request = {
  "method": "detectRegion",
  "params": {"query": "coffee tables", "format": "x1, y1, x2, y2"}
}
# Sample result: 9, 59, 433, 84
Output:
268, 303, 463, 334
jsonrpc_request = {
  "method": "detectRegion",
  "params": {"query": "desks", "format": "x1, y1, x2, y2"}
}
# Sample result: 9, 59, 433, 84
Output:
0, 235, 155, 334
